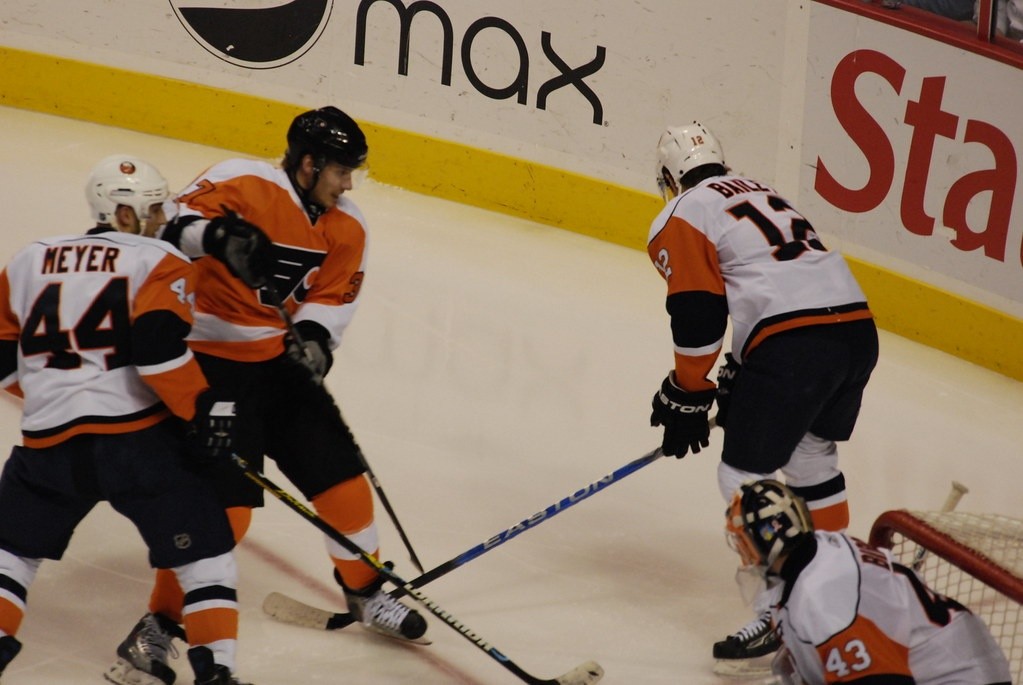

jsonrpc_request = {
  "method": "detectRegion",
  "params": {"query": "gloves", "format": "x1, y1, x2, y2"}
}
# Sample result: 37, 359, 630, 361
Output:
188, 387, 244, 486
650, 368, 718, 459
202, 217, 276, 291
283, 320, 334, 394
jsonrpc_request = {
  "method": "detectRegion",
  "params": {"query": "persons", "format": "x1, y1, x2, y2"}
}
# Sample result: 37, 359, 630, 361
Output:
650, 117, 880, 678
724, 478, 1014, 685
101, 105, 431, 685
0, 155, 257, 685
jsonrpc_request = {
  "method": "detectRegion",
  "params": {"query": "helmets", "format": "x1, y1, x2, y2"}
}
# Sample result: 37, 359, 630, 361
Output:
283, 106, 368, 178
724, 479, 816, 579
85, 154, 169, 231
655, 120, 732, 196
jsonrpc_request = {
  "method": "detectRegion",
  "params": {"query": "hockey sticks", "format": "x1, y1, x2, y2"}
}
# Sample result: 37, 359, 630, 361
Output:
226, 449, 608, 684
269, 281, 427, 577
712, 479, 969, 679
260, 417, 720, 632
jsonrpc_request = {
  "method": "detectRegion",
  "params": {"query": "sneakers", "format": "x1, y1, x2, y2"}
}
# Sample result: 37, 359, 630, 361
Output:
188, 645, 239, 685
333, 559, 433, 646
0, 635, 23, 677
102, 611, 188, 685
711, 607, 786, 677
